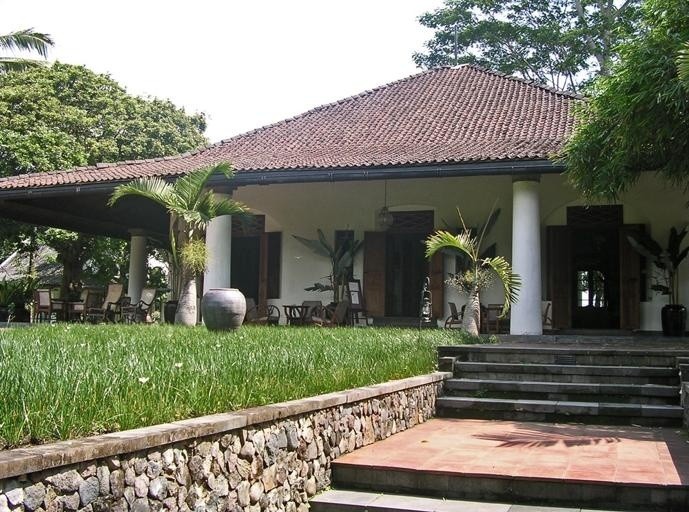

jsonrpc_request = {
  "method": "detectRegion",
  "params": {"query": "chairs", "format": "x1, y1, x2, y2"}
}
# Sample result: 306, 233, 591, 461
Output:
444, 301, 464, 330
31, 282, 157, 324
245, 297, 370, 329
543, 302, 556, 332
483, 303, 507, 333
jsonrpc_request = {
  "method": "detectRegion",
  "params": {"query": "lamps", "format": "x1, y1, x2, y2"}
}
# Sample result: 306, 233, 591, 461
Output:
376, 177, 394, 227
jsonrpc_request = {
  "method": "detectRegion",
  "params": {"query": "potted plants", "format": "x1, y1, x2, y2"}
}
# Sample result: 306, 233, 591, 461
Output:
625, 221, 689, 337
291, 224, 370, 318
